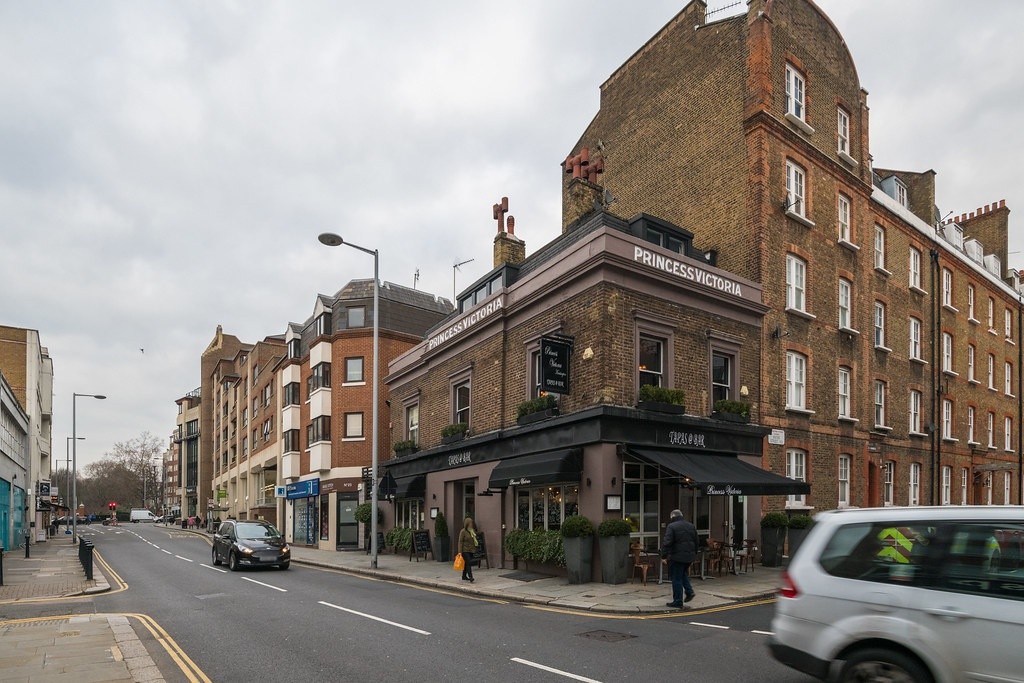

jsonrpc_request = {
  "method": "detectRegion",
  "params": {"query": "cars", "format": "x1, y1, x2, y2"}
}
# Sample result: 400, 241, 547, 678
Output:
77, 516, 93, 525
154, 515, 177, 523
50, 516, 78, 525
211, 520, 292, 571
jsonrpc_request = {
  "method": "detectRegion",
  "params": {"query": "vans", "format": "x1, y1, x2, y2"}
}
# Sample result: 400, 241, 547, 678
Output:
128, 507, 158, 523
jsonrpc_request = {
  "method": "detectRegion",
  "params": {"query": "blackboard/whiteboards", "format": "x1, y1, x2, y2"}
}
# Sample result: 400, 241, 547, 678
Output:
471, 532, 490, 569
409, 529, 435, 562
366, 531, 386, 555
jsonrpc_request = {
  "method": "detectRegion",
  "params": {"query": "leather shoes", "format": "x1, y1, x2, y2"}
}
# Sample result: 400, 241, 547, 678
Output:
684, 592, 694, 602
666, 600, 682, 607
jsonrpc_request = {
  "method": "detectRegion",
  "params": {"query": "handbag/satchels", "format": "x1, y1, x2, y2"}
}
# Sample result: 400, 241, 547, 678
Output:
454, 553, 464, 570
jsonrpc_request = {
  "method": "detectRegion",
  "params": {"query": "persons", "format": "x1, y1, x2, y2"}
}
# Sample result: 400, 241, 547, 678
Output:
458, 517, 477, 581
195, 515, 201, 529
660, 509, 700, 607
187, 515, 195, 529
52, 518, 60, 534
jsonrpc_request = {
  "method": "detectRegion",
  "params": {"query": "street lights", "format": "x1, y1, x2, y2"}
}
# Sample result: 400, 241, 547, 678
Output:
55, 458, 73, 534
64, 435, 86, 534
71, 391, 107, 541
315, 231, 382, 570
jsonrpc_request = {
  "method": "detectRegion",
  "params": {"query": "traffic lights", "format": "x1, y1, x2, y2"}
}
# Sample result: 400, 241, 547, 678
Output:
109, 503, 112, 509
113, 502, 116, 510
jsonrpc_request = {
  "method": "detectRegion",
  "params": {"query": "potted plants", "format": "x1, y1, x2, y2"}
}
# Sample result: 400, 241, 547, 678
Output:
598, 518, 632, 584
759, 513, 787, 567
433, 512, 451, 563
558, 513, 596, 583
789, 515, 817, 561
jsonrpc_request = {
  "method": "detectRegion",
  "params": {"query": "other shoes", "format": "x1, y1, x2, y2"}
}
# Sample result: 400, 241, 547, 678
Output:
461, 575, 469, 580
469, 577, 474, 581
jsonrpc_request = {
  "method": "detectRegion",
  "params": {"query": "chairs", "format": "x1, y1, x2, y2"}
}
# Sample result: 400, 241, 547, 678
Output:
629, 534, 759, 587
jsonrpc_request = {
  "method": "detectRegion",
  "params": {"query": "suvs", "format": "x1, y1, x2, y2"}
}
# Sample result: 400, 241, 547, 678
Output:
763, 503, 1024, 683
